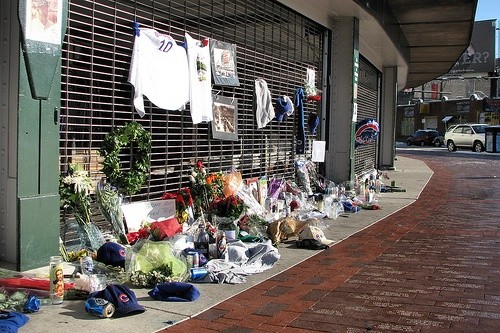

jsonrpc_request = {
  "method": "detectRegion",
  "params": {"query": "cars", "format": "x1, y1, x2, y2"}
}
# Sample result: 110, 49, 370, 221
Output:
432, 135, 444, 147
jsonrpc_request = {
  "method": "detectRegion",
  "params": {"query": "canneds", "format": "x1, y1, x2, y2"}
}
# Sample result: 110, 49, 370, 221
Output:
187, 267, 208, 279
187, 251, 199, 269
80, 256, 93, 275
85, 297, 115, 318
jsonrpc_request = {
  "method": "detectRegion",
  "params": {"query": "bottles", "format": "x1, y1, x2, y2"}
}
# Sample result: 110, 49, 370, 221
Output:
216, 229, 228, 259
194, 224, 210, 262
375, 177, 381, 194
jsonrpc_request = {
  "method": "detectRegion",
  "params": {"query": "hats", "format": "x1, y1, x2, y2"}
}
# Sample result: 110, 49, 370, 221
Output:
308, 113, 319, 134
97, 242, 131, 270
283, 95, 294, 121
89, 284, 147, 317
276, 98, 289, 121
183, 248, 209, 267
299, 226, 336, 245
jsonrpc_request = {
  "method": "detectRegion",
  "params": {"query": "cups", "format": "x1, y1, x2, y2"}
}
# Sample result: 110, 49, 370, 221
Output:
49, 256, 63, 305
80, 256, 94, 274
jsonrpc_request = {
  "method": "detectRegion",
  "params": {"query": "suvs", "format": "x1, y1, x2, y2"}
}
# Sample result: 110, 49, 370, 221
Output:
406, 129, 439, 145
445, 123, 490, 151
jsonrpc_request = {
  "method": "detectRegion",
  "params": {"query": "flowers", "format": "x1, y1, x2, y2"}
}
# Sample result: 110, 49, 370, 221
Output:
125, 218, 189, 288
162, 160, 270, 242
59, 161, 105, 252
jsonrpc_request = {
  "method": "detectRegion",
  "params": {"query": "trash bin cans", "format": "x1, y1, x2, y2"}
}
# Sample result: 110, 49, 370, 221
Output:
484, 125, 500, 153
